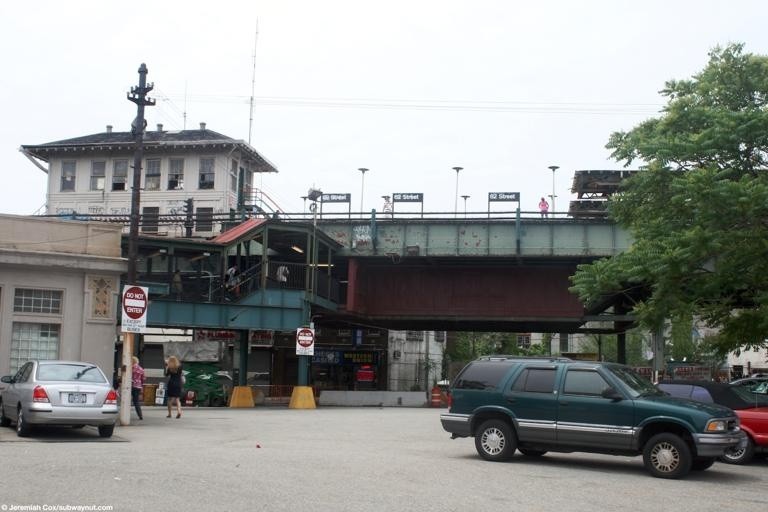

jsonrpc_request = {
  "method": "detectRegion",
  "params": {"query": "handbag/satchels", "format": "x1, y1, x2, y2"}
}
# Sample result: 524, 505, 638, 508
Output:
180, 375, 185, 385
283, 268, 289, 278
225, 274, 230, 282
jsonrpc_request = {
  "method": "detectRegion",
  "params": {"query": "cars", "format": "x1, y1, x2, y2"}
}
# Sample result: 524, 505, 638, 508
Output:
0, 359, 119, 438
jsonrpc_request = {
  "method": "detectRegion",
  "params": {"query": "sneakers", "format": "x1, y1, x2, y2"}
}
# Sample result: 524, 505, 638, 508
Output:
176, 413, 180, 418
166, 415, 171, 417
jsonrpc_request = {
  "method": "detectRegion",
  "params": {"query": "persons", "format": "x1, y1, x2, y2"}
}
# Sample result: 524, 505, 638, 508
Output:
224, 262, 239, 302
276, 264, 290, 288
171, 269, 184, 301
539, 197, 549, 218
132, 356, 146, 420
163, 356, 184, 418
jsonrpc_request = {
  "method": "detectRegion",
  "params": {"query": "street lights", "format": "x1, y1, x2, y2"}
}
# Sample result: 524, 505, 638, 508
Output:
548, 165, 560, 218
300, 196, 309, 219
452, 167, 470, 218
357, 168, 370, 218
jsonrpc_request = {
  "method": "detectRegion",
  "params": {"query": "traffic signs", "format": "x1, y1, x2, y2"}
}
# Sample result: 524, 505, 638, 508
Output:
120, 284, 149, 333
295, 327, 316, 356
